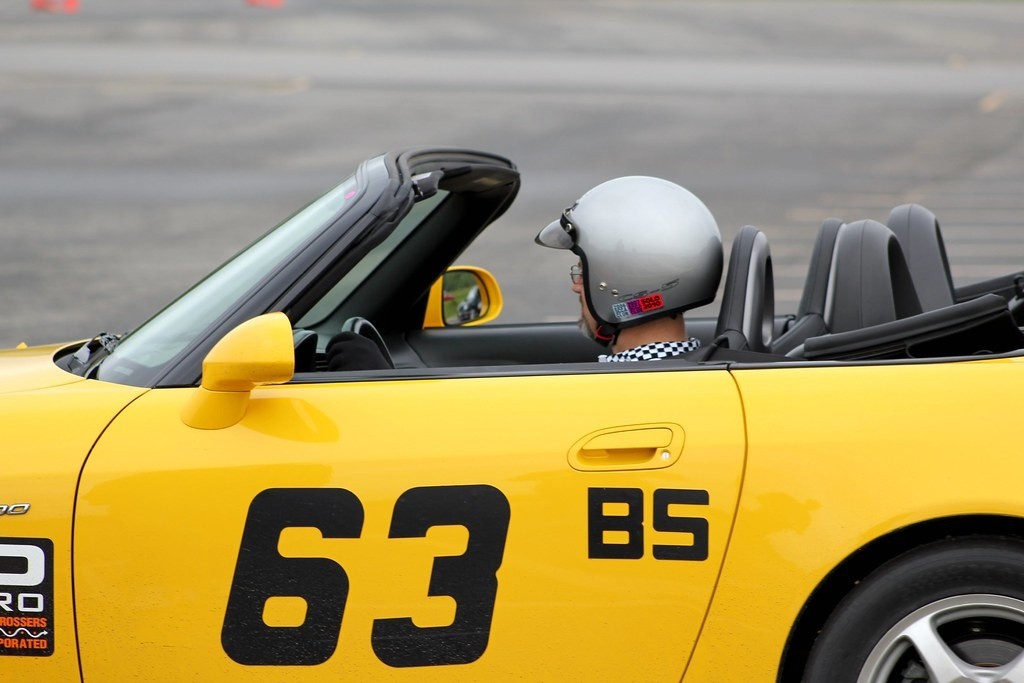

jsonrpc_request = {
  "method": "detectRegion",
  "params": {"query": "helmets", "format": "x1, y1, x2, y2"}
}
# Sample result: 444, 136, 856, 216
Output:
535, 176, 724, 327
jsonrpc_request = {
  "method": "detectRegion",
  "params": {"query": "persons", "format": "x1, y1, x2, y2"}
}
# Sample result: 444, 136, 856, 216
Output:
533, 173, 724, 363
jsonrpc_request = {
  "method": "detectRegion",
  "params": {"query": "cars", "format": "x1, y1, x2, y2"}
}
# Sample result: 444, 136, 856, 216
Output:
0, 146, 1024, 683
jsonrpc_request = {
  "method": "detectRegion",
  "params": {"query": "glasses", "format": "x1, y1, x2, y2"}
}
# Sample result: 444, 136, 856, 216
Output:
569, 265, 584, 282
